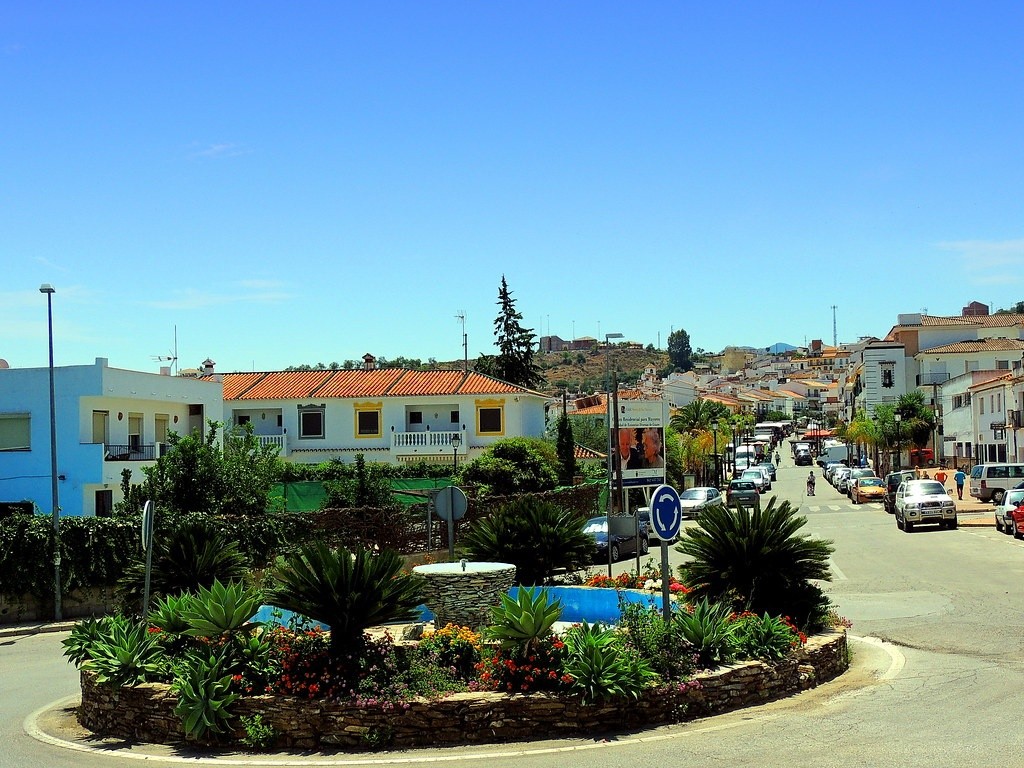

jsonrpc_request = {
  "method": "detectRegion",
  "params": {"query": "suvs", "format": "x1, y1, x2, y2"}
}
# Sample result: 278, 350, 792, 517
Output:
893, 480, 957, 532
794, 443, 813, 467
879, 470, 920, 514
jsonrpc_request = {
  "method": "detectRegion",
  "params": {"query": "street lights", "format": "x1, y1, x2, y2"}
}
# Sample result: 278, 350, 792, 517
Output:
731, 419, 738, 480
736, 419, 741, 446
40, 284, 63, 623
815, 417, 822, 457
893, 408, 901, 470
451, 432, 461, 476
712, 418, 719, 490
605, 332, 624, 517
743, 420, 750, 467
871, 414, 880, 477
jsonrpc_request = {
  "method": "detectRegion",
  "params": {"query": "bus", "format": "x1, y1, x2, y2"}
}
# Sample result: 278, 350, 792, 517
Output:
741, 419, 794, 458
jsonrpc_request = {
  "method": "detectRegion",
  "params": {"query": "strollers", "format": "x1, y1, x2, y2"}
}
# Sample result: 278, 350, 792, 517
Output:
807, 481, 815, 496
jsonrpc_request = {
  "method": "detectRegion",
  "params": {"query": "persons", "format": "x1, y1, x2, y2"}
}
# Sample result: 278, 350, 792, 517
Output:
642, 428, 664, 468
612, 428, 642, 470
915, 466, 920, 477
954, 467, 966, 500
934, 468, 948, 486
807, 471, 815, 491
768, 451, 771, 462
779, 437, 782, 447
921, 470, 929, 478
775, 451, 779, 466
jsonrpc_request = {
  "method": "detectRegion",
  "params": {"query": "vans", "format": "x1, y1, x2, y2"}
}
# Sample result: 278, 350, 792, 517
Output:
846, 465, 875, 498
731, 446, 758, 476
970, 462, 1024, 503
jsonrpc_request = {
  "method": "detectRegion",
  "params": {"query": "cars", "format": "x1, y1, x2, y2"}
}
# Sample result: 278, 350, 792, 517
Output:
851, 477, 888, 504
747, 466, 772, 490
632, 507, 681, 545
758, 462, 777, 482
726, 479, 761, 510
580, 517, 649, 563
738, 469, 767, 494
993, 488, 1024, 534
910, 448, 934, 466
1011, 497, 1024, 538
823, 460, 850, 494
679, 488, 722, 517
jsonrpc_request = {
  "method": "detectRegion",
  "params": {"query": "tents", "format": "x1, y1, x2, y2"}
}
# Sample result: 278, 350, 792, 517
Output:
804, 430, 830, 436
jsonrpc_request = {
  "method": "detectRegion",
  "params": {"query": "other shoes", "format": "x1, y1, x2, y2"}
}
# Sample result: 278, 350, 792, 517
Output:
959, 496, 962, 499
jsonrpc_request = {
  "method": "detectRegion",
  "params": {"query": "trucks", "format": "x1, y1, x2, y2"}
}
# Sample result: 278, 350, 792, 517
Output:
816, 443, 861, 467
